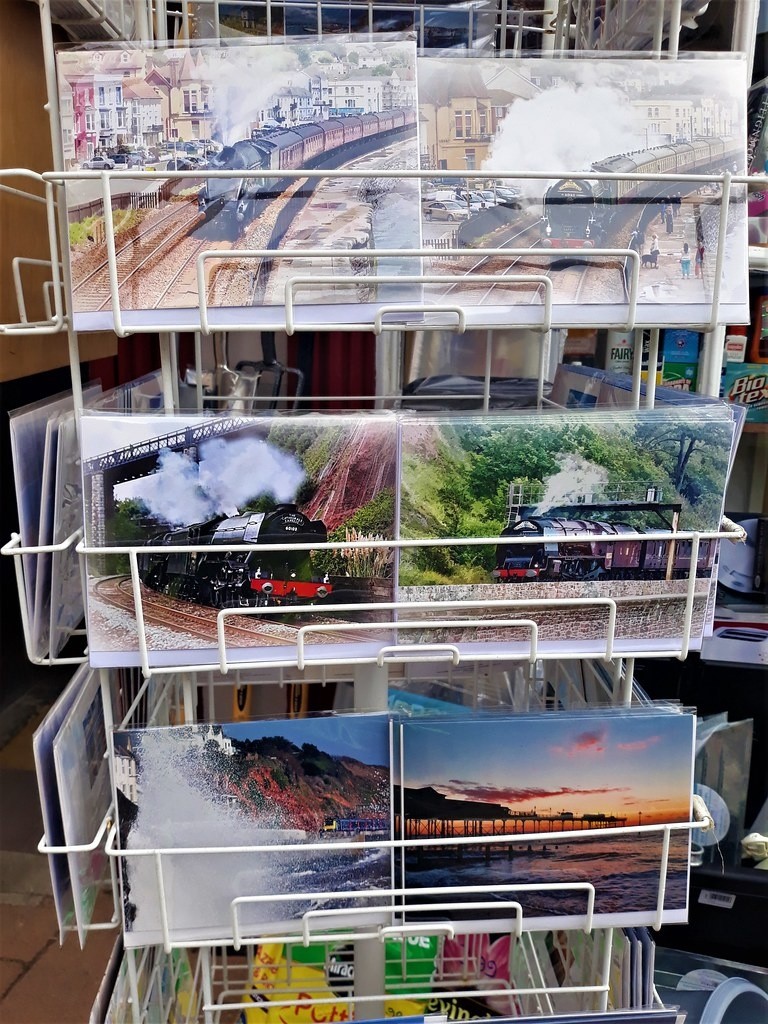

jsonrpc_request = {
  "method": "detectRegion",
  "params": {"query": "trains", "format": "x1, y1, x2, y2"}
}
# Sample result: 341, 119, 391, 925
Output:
131, 501, 331, 622
538, 130, 744, 267
489, 479, 721, 581
194, 104, 416, 244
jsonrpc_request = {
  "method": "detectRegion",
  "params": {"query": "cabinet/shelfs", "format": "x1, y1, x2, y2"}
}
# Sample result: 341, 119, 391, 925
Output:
0, 0, 763, 1024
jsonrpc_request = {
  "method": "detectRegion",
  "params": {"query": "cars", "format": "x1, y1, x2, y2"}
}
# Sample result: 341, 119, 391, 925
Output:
484, 187, 520, 203
109, 137, 225, 164
421, 180, 436, 202
82, 155, 116, 170
421, 199, 469, 223
472, 190, 508, 205
450, 192, 488, 210
